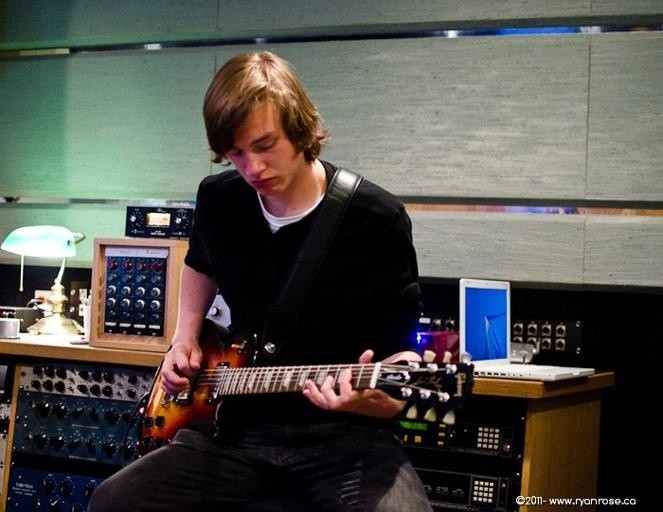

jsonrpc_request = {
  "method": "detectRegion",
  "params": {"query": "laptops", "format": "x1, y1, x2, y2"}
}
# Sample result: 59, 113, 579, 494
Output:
458, 277, 595, 383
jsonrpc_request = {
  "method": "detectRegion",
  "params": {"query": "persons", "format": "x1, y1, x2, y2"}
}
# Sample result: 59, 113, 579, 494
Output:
88, 51, 429, 511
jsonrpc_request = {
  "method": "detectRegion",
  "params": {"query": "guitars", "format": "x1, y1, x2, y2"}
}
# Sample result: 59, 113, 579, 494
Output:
135, 318, 475, 455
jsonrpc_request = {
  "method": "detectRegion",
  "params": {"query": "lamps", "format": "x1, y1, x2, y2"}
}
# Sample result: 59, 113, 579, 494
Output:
0, 222, 87, 338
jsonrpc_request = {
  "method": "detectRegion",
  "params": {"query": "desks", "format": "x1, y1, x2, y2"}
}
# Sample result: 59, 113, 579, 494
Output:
0, 332, 616, 512
